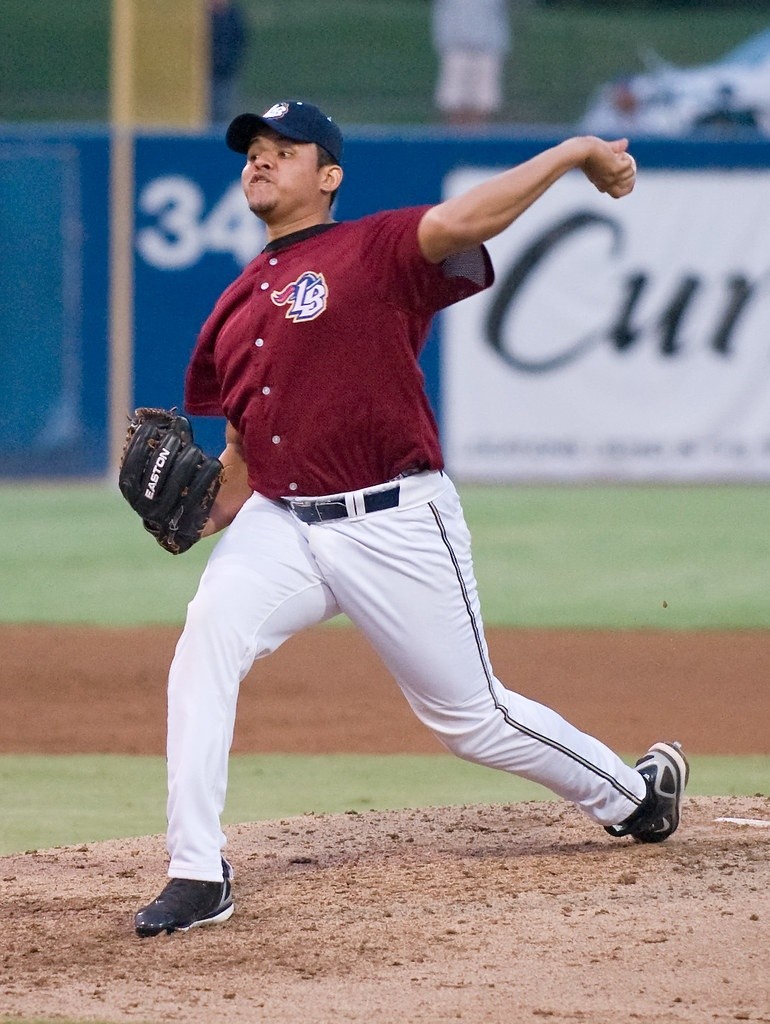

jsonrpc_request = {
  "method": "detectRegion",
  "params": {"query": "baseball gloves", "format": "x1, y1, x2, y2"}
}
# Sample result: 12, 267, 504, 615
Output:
117, 407, 226, 556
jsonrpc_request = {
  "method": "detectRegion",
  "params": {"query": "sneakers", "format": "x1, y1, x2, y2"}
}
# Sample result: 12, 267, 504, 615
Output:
134, 859, 239, 939
601, 741, 692, 843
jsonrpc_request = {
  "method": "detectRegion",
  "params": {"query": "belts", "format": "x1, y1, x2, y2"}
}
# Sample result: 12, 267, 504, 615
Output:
270, 487, 400, 524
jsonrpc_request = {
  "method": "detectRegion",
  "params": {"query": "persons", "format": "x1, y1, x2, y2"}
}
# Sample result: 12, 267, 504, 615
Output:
431, 0, 518, 124
116, 100, 689, 938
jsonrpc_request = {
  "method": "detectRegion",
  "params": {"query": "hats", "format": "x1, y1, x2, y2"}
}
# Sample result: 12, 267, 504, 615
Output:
226, 101, 343, 164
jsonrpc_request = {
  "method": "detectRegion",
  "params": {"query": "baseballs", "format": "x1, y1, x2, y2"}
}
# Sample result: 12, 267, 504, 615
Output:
617, 152, 637, 175
